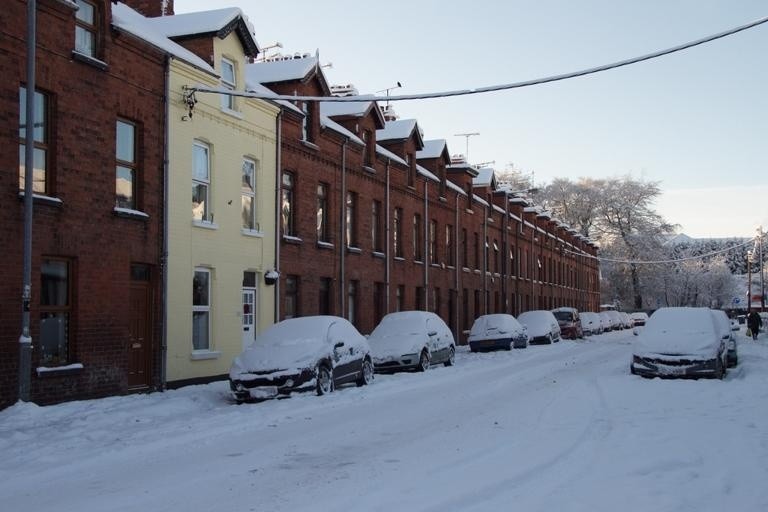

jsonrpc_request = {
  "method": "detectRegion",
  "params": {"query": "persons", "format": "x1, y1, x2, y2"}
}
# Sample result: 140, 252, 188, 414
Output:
747, 308, 763, 340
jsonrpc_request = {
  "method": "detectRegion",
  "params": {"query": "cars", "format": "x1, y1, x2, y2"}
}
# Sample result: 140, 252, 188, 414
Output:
631, 305, 740, 379
364, 310, 457, 375
228, 314, 374, 404
39, 315, 67, 363
466, 304, 650, 353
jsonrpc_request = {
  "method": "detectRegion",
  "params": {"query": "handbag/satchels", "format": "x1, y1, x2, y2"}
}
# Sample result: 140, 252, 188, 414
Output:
745, 328, 753, 337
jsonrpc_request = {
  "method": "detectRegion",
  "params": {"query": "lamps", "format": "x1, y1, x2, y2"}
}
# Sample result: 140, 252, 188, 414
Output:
265, 263, 279, 278
